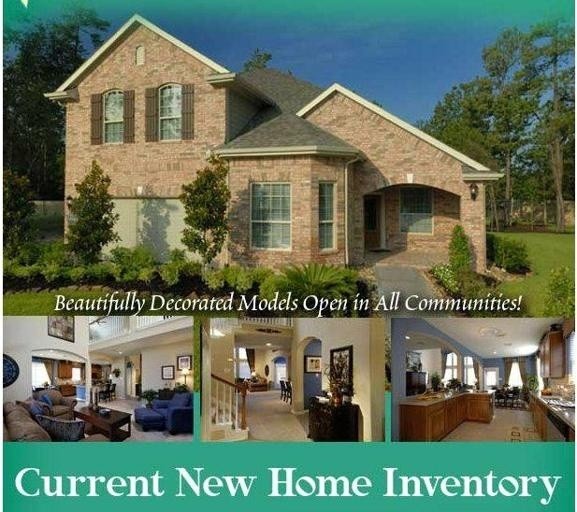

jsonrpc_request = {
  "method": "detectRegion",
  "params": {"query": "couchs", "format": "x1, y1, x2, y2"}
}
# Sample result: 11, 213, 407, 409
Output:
3, 399, 110, 442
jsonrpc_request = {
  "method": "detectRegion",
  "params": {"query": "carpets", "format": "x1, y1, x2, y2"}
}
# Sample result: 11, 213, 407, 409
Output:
507, 425, 541, 442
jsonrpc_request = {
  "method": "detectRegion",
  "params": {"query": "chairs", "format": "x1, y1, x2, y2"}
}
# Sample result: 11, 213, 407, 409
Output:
90, 380, 117, 402
29, 389, 77, 422
279, 380, 293, 404
134, 387, 194, 433
246, 378, 268, 391
492, 385, 520, 409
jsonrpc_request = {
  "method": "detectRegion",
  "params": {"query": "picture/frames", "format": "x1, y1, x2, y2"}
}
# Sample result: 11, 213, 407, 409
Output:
48, 315, 75, 343
176, 354, 192, 371
330, 345, 354, 396
160, 365, 175, 381
304, 354, 322, 374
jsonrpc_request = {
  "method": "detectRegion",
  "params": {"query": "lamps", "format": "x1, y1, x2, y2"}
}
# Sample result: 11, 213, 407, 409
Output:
180, 368, 190, 385
468, 181, 479, 200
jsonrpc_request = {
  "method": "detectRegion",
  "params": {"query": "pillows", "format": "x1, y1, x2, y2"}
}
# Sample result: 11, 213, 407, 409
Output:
16, 400, 85, 441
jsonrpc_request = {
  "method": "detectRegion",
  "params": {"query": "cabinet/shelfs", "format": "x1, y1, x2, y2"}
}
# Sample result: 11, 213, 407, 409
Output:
307, 395, 359, 442
539, 329, 564, 378
59, 364, 72, 378
529, 390, 566, 441
400, 388, 493, 441
406, 371, 426, 396
